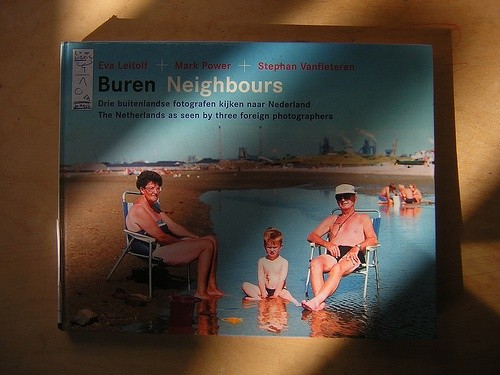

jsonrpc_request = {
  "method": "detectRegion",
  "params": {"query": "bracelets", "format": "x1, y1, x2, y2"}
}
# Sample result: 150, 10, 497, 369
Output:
356, 244, 361, 251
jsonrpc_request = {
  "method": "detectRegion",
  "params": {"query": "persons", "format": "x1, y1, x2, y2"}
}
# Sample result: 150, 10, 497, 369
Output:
126, 170, 225, 300
377, 182, 422, 204
301, 184, 379, 311
242, 227, 301, 306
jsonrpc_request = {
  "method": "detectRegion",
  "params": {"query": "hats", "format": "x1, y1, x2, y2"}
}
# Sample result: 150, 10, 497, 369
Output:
334, 183, 358, 196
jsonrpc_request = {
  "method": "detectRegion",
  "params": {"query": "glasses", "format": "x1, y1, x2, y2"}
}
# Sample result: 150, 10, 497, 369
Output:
335, 193, 353, 201
142, 184, 164, 193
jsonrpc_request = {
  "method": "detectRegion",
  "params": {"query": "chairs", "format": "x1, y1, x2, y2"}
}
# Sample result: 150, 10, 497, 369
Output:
106, 191, 193, 300
304, 208, 382, 310
